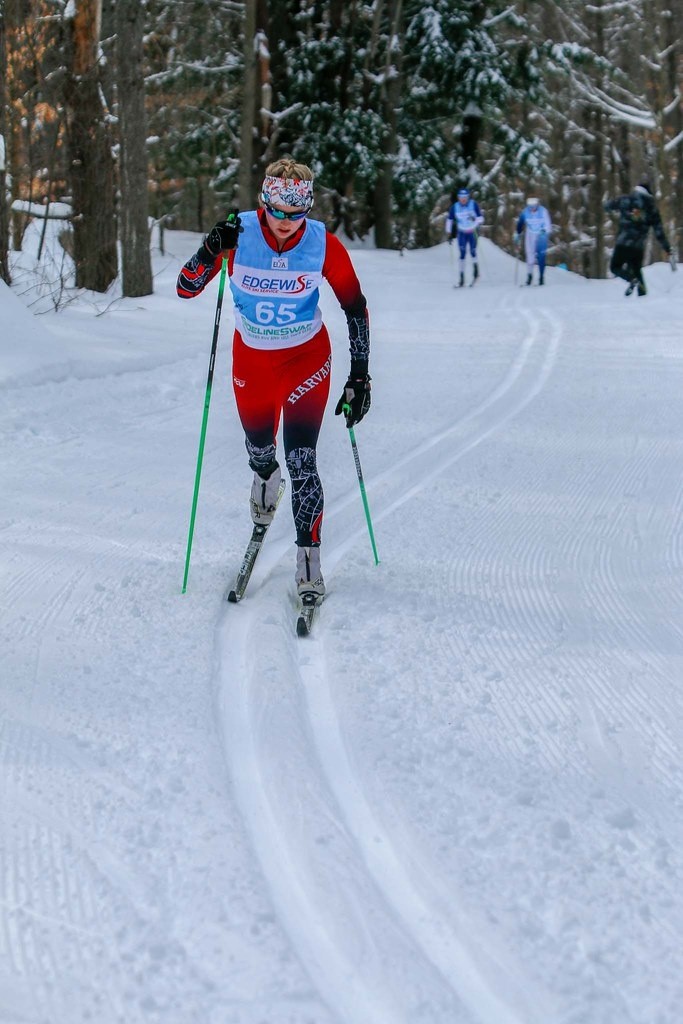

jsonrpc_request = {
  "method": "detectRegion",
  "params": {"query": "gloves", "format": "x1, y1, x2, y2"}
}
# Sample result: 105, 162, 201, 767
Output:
203, 216, 244, 256
335, 374, 371, 428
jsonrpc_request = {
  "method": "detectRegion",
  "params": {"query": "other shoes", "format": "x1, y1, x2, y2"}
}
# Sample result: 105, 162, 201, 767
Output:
626, 278, 638, 296
637, 286, 646, 295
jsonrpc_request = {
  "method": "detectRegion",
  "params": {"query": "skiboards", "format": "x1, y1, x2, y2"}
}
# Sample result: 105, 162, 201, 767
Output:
226, 478, 319, 636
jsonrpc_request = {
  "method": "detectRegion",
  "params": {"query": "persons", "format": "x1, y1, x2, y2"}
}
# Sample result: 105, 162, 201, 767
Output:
607, 178, 673, 296
445, 188, 486, 286
176, 157, 371, 599
513, 194, 553, 285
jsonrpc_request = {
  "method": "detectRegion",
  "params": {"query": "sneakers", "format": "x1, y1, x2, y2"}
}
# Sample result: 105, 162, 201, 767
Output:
296, 546, 327, 599
250, 461, 281, 524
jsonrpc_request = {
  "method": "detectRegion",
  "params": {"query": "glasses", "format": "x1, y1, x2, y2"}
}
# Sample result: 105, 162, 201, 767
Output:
264, 202, 313, 221
